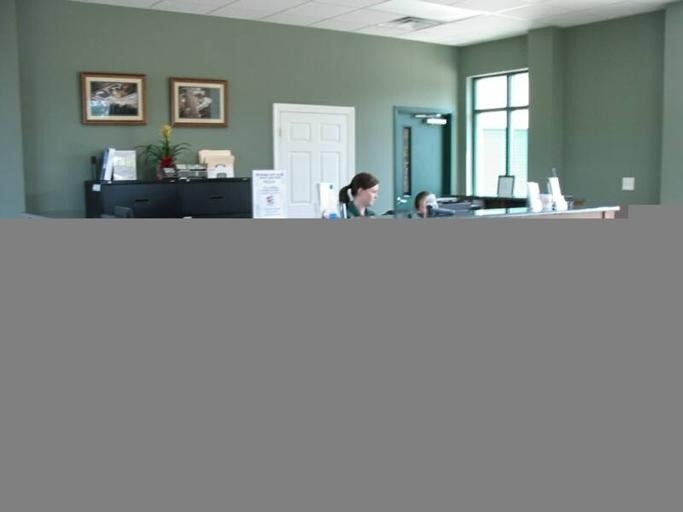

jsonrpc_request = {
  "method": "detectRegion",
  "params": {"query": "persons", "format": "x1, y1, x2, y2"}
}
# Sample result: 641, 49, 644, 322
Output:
340, 171, 380, 219
410, 191, 439, 217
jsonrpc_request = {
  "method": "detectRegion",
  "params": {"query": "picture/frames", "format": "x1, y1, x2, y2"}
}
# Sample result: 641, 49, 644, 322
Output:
169, 78, 228, 127
81, 72, 146, 125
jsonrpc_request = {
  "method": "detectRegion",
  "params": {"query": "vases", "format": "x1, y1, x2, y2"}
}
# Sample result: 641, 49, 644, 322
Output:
158, 157, 174, 180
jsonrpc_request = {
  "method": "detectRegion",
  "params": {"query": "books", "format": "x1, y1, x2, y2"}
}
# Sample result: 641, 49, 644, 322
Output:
203, 155, 234, 179
198, 149, 230, 165
112, 150, 137, 181
100, 146, 115, 181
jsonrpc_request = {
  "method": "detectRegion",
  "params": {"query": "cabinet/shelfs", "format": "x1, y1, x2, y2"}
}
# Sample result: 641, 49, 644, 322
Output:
84, 177, 252, 218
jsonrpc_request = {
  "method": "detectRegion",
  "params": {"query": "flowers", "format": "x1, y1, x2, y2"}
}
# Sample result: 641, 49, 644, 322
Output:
134, 126, 192, 175
